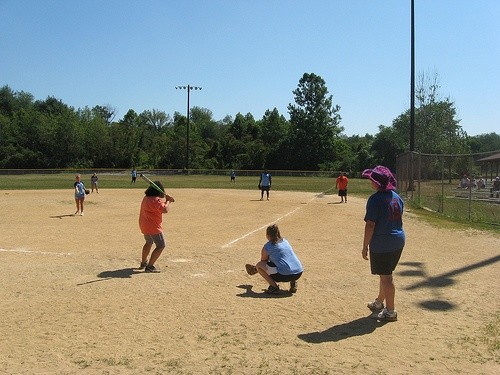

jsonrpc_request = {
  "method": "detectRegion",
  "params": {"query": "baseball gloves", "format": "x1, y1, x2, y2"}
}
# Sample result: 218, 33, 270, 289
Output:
258, 185, 262, 190
85, 189, 90, 195
245, 264, 259, 275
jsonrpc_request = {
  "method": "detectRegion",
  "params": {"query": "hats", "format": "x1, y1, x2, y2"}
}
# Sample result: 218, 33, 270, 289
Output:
362, 166, 397, 191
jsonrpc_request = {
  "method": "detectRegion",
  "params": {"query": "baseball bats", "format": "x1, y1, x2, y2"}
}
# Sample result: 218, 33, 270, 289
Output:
140, 174, 175, 202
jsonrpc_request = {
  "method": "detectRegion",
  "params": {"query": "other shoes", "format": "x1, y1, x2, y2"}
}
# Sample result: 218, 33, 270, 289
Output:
265, 285, 280, 295
145, 265, 160, 273
141, 261, 149, 268
289, 287, 297, 293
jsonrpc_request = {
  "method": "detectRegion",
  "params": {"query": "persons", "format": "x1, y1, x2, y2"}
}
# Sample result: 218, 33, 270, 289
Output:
91, 172, 98, 193
361, 165, 406, 321
336, 172, 348, 203
258, 169, 272, 201
74, 175, 87, 216
490, 177, 500, 198
131, 165, 137, 183
138, 181, 174, 273
459, 174, 486, 190
230, 168, 236, 183
256, 224, 303, 294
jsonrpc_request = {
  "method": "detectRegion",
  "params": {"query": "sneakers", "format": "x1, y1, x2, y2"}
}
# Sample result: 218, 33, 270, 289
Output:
368, 298, 385, 311
372, 307, 397, 321
